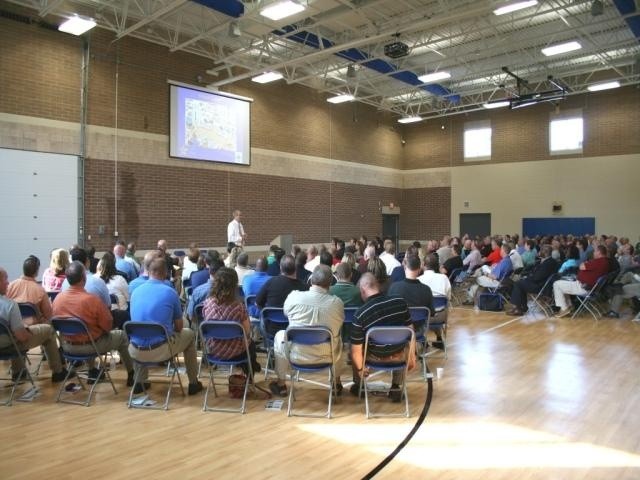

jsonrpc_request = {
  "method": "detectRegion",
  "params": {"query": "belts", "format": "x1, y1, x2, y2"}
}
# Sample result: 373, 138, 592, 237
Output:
368, 349, 406, 361
129, 339, 167, 351
65, 336, 103, 346
0, 342, 19, 351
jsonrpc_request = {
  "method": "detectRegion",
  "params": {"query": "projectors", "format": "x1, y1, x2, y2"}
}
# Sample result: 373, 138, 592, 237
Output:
384, 42, 410, 58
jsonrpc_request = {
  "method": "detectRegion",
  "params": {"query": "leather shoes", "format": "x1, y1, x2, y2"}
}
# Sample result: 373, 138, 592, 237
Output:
463, 277, 476, 283
602, 310, 620, 318
521, 304, 528, 312
506, 307, 523, 316
389, 386, 404, 403
189, 381, 203, 396
127, 369, 135, 386
269, 381, 288, 397
88, 368, 105, 385
350, 384, 365, 398
134, 382, 151, 395
463, 300, 474, 305
331, 383, 343, 396
52, 368, 77, 383
12, 368, 28, 380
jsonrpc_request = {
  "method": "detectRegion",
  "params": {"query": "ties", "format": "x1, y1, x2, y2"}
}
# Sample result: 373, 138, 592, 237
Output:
238, 223, 243, 237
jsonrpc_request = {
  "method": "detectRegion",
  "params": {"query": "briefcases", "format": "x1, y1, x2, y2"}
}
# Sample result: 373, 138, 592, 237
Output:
478, 293, 503, 311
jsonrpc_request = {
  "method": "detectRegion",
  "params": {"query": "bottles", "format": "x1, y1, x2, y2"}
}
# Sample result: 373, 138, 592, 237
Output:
467, 288, 471, 303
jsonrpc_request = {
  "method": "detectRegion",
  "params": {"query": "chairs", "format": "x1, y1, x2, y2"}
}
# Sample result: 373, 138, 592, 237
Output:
487, 267, 616, 324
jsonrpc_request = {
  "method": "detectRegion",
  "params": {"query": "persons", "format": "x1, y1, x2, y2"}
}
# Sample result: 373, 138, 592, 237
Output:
1, 237, 640, 400
226, 209, 249, 254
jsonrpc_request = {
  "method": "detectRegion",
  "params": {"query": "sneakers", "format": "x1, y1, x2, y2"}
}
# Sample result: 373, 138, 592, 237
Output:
554, 306, 571, 318
569, 303, 575, 311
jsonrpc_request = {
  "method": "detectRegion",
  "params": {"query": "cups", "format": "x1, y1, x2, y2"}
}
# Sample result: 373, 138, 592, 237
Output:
110, 358, 116, 371
437, 368, 444, 380
